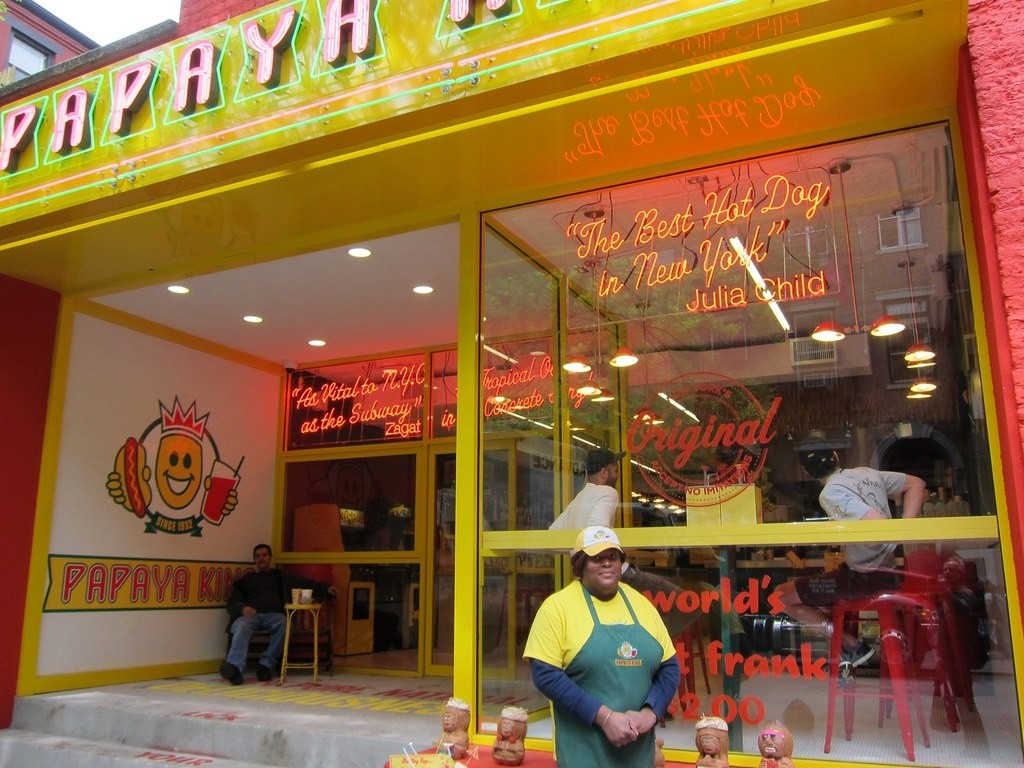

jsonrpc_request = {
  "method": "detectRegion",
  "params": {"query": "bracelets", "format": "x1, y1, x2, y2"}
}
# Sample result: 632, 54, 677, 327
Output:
602, 711, 613, 729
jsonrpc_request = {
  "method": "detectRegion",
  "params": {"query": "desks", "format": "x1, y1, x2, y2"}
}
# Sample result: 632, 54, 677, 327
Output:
383, 742, 700, 768
275, 602, 323, 686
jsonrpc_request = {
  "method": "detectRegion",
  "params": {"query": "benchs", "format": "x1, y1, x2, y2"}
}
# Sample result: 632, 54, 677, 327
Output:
225, 595, 336, 677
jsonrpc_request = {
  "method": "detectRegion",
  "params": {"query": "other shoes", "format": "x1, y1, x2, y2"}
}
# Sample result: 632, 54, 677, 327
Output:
220, 659, 242, 685
836, 641, 876, 668
255, 665, 270, 682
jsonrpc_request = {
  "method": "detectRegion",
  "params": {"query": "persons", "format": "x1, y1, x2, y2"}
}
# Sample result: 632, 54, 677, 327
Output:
492, 706, 527, 764
435, 696, 470, 760
758, 721, 795, 768
219, 544, 341, 686
523, 525, 680, 768
932, 557, 991, 751
696, 717, 730, 768
549, 448, 702, 721
774, 437, 926, 670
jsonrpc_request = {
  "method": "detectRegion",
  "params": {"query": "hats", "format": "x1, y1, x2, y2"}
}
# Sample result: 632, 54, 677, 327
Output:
574, 524, 626, 556
586, 446, 628, 473
797, 437, 832, 462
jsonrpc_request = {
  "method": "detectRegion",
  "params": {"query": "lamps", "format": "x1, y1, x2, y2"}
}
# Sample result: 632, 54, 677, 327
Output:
561, 156, 941, 402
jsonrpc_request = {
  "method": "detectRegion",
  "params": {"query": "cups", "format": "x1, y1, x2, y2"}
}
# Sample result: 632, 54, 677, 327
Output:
302, 590, 313, 603
291, 589, 301, 605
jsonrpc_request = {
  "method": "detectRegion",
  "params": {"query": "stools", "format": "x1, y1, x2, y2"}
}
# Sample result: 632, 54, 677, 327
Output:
657, 545, 974, 762
